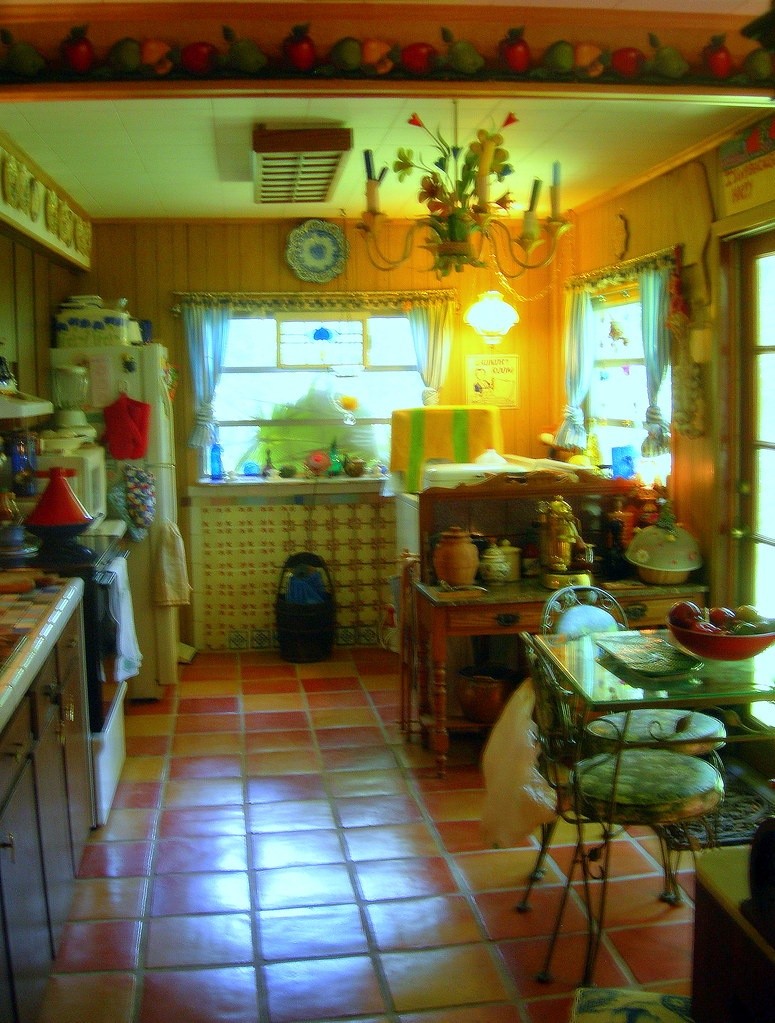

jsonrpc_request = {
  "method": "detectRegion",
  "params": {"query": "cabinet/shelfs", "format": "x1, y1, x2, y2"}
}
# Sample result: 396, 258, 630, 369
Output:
0, 593, 97, 1023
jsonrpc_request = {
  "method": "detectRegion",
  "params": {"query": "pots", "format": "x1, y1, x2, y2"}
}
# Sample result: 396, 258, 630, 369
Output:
25, 513, 105, 539
0, 524, 42, 567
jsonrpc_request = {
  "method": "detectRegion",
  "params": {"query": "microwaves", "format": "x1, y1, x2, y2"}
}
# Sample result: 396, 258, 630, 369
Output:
7, 445, 107, 531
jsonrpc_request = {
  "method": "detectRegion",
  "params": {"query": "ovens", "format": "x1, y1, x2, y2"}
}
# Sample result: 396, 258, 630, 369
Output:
69, 550, 127, 828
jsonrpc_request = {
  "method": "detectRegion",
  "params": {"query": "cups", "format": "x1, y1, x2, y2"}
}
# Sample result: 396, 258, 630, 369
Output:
612, 446, 636, 480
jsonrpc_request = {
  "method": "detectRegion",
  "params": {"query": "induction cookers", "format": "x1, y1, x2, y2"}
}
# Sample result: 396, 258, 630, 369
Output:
0, 535, 130, 585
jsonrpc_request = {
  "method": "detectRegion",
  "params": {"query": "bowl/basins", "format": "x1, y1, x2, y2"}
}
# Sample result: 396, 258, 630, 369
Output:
624, 525, 704, 571
39, 437, 82, 452
637, 567, 690, 584
665, 617, 775, 661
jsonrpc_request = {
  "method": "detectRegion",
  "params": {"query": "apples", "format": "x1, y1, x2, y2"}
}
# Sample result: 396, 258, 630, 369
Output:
670, 601, 775, 635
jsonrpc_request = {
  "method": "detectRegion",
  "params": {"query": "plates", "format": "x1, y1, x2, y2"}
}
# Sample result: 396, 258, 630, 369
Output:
596, 636, 704, 681
284, 220, 350, 283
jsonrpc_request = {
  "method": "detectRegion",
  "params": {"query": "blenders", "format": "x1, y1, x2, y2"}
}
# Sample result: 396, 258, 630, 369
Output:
48, 365, 97, 439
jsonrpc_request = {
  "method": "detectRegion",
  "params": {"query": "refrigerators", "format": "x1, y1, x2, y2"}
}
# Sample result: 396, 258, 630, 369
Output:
48, 344, 178, 699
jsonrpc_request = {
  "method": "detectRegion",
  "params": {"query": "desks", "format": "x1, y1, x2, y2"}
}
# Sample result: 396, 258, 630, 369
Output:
525, 633, 774, 748
410, 574, 710, 780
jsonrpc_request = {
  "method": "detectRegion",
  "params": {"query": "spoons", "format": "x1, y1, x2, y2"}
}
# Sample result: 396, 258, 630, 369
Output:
440, 580, 488, 592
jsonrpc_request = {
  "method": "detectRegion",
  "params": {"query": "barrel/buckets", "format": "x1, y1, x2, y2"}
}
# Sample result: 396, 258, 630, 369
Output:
455, 665, 512, 722
274, 552, 336, 662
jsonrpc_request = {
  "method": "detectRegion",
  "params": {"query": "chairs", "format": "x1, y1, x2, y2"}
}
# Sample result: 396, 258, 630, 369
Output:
539, 585, 725, 898
514, 631, 726, 994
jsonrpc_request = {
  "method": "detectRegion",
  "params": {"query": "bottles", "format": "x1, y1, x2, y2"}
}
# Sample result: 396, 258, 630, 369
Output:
582, 495, 660, 581
209, 443, 223, 479
422, 521, 540, 585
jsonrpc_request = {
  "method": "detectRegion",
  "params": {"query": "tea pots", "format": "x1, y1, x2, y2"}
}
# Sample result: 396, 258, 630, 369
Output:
336, 454, 367, 476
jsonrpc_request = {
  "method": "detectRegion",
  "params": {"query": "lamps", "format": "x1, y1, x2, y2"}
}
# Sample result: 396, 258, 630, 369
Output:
355, 100, 577, 279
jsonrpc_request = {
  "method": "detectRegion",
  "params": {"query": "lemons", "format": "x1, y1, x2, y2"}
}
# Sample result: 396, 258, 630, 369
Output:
339, 396, 358, 410
